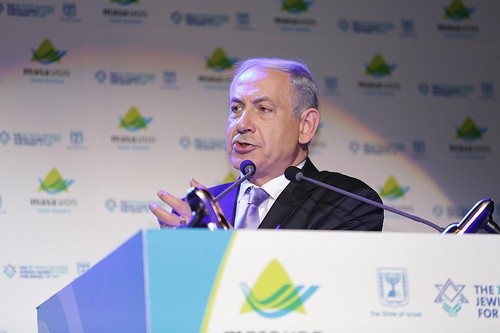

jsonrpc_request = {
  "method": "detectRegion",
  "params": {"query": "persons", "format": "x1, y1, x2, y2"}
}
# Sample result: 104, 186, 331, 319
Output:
149, 58, 385, 231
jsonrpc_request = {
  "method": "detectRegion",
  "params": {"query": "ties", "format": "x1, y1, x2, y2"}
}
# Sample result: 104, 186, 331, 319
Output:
238, 187, 270, 228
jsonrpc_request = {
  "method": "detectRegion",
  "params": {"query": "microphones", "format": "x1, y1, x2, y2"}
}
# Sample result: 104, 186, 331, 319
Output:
284, 166, 446, 233
188, 160, 256, 227
